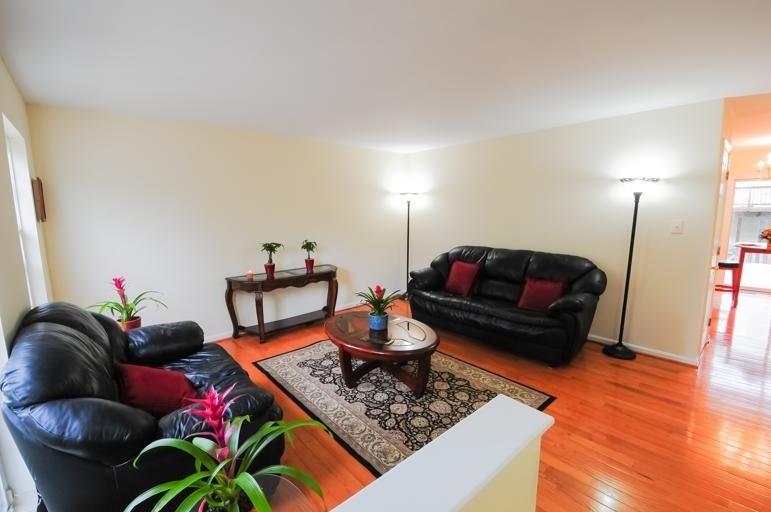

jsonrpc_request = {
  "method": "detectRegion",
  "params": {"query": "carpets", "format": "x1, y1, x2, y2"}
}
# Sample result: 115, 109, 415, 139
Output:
252, 338, 557, 479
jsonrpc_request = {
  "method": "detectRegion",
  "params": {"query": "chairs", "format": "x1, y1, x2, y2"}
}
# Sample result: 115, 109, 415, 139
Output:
714, 245, 739, 302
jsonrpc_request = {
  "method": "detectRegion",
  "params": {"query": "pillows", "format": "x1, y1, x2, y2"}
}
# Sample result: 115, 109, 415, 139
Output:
114, 363, 201, 414
518, 277, 563, 310
445, 260, 479, 296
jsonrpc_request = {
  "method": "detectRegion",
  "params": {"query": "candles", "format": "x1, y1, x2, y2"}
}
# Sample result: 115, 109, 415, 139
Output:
247, 270, 253, 279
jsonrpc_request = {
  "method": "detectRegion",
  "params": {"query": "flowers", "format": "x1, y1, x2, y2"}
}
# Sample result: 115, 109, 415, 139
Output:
124, 381, 335, 511
760, 228, 771, 244
354, 285, 403, 315
85, 276, 169, 329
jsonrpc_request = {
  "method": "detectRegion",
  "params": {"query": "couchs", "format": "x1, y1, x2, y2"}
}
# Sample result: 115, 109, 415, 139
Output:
0, 302, 285, 512
406, 246, 607, 367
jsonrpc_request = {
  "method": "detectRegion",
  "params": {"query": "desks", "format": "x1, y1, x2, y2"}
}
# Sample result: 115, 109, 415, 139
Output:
732, 242, 771, 307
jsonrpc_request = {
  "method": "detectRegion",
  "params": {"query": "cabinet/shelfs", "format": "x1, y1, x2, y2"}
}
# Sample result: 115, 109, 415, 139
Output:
224, 264, 338, 343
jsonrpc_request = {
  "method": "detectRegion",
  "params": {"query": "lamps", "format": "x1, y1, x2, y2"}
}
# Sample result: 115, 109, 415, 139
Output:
602, 173, 658, 359
394, 185, 425, 301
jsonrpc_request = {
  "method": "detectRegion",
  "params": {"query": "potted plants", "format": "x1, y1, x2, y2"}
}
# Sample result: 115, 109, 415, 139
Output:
301, 240, 318, 274
261, 241, 284, 280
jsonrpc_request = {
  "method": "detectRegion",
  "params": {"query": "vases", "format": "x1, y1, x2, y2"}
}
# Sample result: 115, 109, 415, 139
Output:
766, 243, 771, 248
115, 317, 142, 331
368, 311, 389, 330
